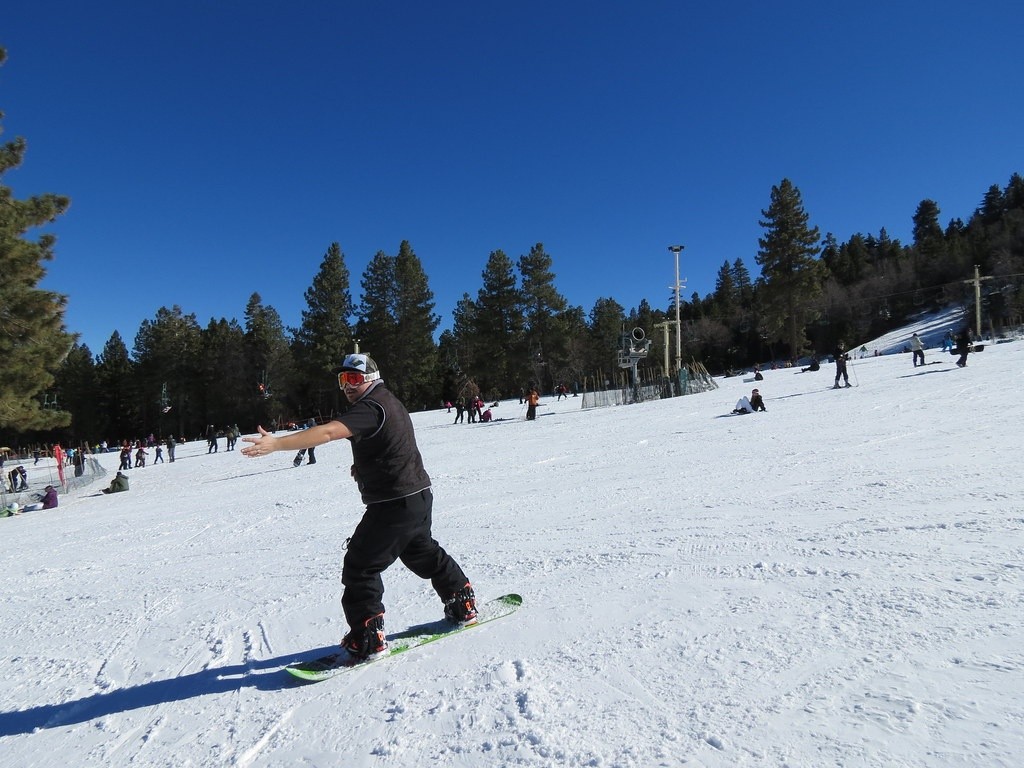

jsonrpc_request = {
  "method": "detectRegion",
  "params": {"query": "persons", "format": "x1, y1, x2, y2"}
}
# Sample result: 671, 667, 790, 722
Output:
733, 388, 766, 413
726, 361, 791, 381
95, 441, 107, 453
105, 472, 129, 493
833, 342, 851, 388
0, 485, 58, 518
802, 356, 819, 373
942, 327, 982, 367
271, 418, 277, 434
447, 395, 499, 424
859, 345, 868, 358
33, 449, 39, 464
117, 433, 175, 470
903, 333, 928, 367
207, 423, 241, 454
520, 387, 539, 420
557, 379, 578, 401
66, 449, 85, 477
288, 418, 318, 467
0, 455, 28, 494
240, 354, 478, 662
256, 382, 272, 400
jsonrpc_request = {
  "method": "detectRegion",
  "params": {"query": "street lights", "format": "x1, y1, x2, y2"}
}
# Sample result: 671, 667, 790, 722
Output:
668, 245, 687, 371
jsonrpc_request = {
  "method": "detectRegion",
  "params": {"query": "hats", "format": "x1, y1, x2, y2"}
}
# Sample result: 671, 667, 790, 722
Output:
331, 354, 380, 380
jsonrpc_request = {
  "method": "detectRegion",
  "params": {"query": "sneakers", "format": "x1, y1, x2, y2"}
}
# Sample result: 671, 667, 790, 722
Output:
340, 613, 388, 657
739, 408, 747, 414
444, 583, 479, 626
733, 410, 740, 414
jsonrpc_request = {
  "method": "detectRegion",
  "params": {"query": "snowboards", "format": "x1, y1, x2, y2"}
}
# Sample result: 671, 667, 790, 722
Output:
284, 593, 523, 682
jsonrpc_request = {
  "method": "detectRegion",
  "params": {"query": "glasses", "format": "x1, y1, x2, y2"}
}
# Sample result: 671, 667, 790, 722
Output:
338, 370, 380, 390
